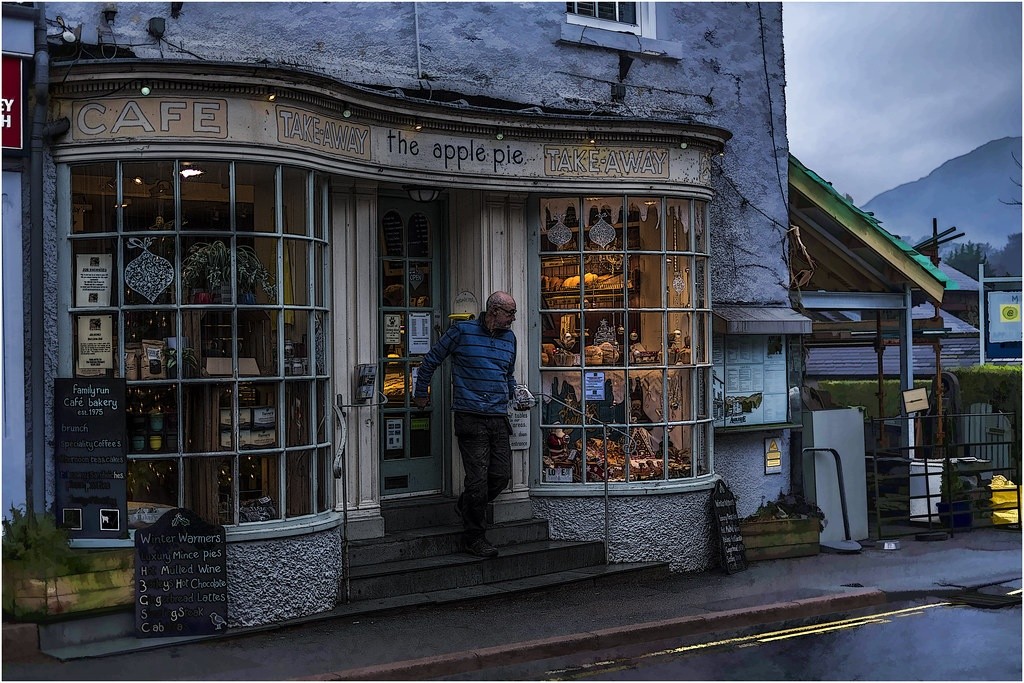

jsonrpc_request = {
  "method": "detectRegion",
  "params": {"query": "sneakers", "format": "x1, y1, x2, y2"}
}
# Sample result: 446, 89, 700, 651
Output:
448, 502, 464, 525
465, 535, 498, 558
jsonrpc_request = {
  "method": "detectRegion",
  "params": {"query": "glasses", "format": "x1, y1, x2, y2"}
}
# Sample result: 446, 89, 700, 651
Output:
498, 306, 517, 316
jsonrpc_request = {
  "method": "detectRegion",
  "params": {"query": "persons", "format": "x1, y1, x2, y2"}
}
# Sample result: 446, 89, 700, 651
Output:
414, 291, 517, 555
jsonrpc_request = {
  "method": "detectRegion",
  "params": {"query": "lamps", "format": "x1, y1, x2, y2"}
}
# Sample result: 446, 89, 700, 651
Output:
106, 172, 124, 190
148, 179, 173, 200
402, 184, 445, 203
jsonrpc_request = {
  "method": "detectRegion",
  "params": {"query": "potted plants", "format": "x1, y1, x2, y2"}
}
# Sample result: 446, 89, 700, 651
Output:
738, 490, 825, 562
2, 494, 136, 617
